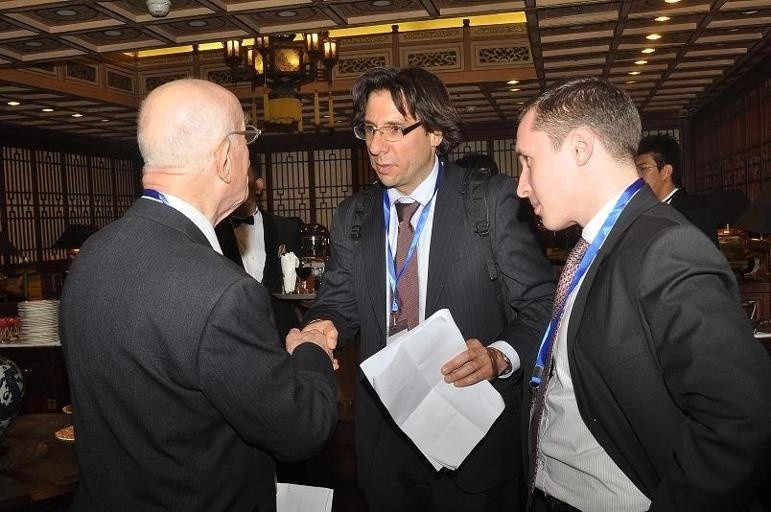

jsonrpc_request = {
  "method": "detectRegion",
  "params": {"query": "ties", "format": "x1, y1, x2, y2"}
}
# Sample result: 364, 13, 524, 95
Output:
389, 199, 420, 336
523, 236, 592, 511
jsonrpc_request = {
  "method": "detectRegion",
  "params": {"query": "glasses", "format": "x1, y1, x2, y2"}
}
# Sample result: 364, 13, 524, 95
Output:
214, 125, 261, 157
355, 121, 421, 142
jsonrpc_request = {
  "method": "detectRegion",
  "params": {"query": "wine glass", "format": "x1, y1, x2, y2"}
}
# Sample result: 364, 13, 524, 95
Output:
295, 257, 311, 296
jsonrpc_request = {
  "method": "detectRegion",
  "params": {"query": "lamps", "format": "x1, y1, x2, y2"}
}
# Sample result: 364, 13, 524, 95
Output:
225, 29, 338, 123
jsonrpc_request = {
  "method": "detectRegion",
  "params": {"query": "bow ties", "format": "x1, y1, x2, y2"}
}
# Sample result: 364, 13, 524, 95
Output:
232, 215, 254, 229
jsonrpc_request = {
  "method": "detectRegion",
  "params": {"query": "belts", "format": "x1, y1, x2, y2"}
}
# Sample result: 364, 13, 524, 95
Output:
532, 487, 580, 511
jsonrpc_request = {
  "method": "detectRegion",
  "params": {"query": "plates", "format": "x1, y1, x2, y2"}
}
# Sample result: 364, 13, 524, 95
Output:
17, 300, 59, 343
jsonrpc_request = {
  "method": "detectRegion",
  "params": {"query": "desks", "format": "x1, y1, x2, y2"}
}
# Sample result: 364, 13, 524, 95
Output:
0, 415, 84, 511
272, 293, 316, 323
0, 342, 63, 353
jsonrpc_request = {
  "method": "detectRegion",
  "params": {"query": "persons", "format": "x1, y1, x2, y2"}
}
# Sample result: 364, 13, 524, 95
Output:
288, 65, 561, 512
55, 77, 340, 512
634, 131, 719, 259
511, 74, 771, 511
207, 147, 314, 361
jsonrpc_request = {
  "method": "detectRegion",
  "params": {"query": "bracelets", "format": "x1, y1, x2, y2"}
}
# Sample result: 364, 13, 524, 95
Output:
483, 344, 501, 382
